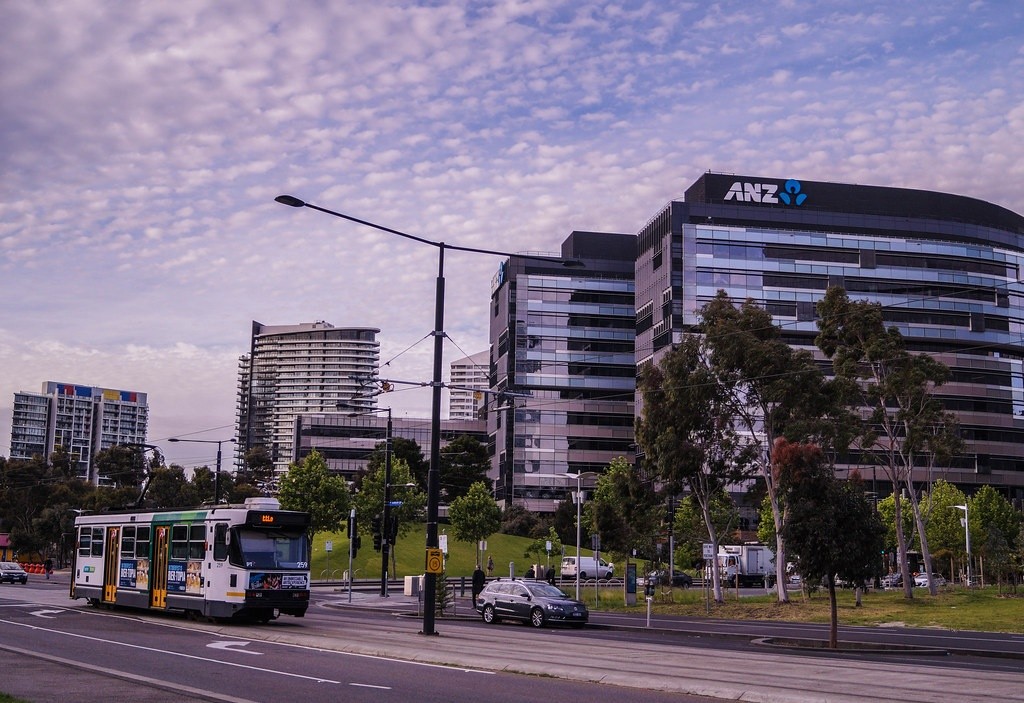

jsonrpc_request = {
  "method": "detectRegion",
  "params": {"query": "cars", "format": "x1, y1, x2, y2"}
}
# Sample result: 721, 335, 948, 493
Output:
882, 574, 914, 588
914, 572, 947, 587
637, 570, 693, 590
0, 561, 28, 585
835, 574, 882, 590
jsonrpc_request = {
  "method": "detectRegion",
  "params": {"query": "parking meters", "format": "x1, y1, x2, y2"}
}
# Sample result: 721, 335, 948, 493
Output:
645, 580, 654, 626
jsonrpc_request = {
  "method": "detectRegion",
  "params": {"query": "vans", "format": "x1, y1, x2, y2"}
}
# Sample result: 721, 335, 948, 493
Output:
561, 557, 616, 580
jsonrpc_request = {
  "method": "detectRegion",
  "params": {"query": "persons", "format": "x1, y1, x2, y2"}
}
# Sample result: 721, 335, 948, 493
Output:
44, 557, 53, 579
486, 556, 494, 577
695, 562, 703, 578
472, 564, 485, 609
548, 565, 556, 587
525, 566, 535, 578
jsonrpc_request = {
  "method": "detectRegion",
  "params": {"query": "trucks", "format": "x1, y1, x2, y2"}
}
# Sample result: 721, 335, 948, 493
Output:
705, 545, 779, 590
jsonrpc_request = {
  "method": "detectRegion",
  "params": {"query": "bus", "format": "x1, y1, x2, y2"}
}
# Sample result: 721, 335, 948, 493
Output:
70, 499, 314, 620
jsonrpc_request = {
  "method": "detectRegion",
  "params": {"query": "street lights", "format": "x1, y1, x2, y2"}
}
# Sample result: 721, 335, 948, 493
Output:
947, 505, 971, 587
275, 194, 584, 630
556, 470, 599, 601
167, 436, 238, 500
335, 403, 415, 596
836, 466, 877, 518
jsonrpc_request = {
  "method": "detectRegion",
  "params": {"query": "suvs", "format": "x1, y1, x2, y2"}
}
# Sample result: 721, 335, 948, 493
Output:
477, 577, 590, 629
